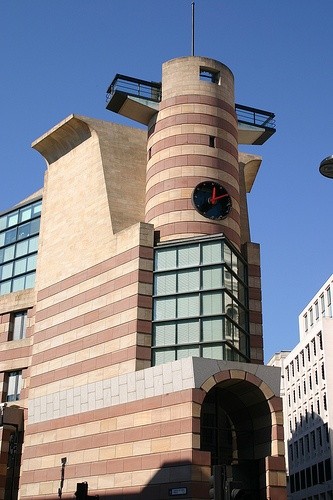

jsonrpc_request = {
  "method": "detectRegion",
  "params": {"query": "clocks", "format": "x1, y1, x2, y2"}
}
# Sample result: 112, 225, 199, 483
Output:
191, 181, 232, 220
